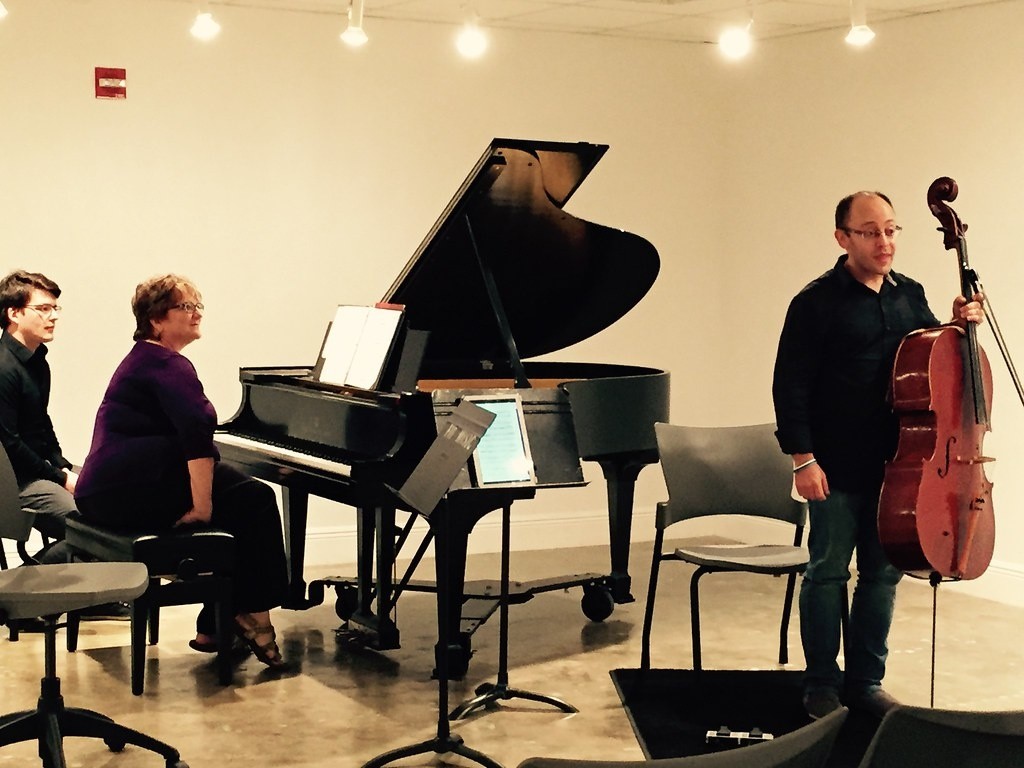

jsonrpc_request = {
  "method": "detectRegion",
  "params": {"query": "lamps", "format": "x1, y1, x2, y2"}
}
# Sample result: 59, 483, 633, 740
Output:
340, 1, 367, 46
845, 7, 875, 48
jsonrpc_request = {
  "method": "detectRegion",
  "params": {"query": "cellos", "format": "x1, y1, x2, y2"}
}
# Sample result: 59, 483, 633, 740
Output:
874, 175, 996, 709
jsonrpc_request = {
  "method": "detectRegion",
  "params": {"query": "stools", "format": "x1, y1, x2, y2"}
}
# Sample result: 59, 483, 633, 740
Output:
66, 508, 232, 695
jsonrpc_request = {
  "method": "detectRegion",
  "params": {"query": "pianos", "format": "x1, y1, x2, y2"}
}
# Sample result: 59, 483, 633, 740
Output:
201, 136, 672, 681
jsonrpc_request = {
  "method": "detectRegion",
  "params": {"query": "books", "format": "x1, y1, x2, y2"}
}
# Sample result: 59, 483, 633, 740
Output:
320, 306, 401, 390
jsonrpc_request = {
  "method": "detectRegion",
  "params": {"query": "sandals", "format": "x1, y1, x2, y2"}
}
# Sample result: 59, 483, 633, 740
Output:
227, 613, 285, 668
188, 633, 219, 653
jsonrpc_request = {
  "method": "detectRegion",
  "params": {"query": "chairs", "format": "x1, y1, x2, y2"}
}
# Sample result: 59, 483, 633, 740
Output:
0, 439, 190, 768
518, 706, 848, 768
856, 703, 1024, 768
641, 423, 809, 671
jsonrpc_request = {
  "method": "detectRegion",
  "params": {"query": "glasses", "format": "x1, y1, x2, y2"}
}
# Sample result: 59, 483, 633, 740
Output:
169, 301, 206, 314
849, 224, 903, 242
25, 303, 62, 314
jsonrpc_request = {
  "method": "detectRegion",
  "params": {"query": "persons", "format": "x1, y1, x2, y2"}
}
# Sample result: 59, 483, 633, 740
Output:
74, 274, 290, 664
0, 270, 132, 620
772, 192, 984, 720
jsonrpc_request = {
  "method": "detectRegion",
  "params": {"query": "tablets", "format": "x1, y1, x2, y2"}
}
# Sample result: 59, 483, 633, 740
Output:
461, 394, 536, 489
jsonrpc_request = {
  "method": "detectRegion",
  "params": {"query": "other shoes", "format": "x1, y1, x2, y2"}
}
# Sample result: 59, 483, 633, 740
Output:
78, 602, 131, 620
802, 692, 842, 721
867, 689, 902, 712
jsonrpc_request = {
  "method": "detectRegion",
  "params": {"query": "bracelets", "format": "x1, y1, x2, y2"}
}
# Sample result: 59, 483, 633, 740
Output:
793, 459, 817, 472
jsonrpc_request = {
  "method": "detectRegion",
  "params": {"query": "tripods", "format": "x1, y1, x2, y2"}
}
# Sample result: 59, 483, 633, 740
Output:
363, 385, 591, 768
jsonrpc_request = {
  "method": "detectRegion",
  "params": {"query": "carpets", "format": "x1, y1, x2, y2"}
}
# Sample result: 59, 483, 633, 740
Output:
609, 667, 875, 768
